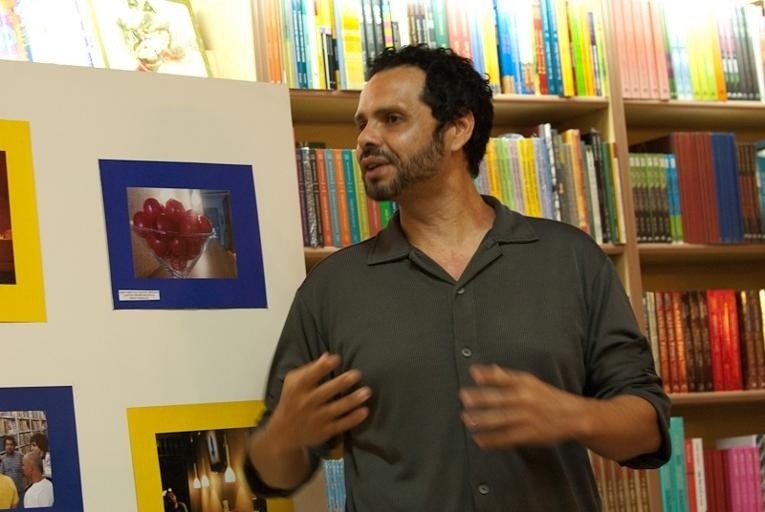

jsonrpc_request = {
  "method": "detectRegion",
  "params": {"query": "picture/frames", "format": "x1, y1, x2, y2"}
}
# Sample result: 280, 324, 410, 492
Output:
205, 430, 226, 472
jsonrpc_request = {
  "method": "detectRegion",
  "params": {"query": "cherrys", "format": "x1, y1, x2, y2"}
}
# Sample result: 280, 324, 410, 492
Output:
133, 197, 213, 271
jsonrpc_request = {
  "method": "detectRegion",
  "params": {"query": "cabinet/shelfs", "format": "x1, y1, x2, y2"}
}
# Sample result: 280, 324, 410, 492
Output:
251, 0, 765, 511
0, 411, 50, 458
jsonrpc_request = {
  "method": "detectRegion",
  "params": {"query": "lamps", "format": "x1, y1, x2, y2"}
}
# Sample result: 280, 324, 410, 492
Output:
191, 443, 236, 489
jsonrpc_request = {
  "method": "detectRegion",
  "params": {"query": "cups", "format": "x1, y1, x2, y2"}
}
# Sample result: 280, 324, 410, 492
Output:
129, 222, 214, 281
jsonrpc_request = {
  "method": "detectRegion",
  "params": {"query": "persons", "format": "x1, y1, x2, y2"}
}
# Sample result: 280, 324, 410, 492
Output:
0, 432, 55, 511
243, 48, 672, 512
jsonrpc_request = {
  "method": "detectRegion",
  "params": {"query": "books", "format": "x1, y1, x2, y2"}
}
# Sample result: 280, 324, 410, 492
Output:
280, 0, 760, 101
642, 289, 765, 392
295, 141, 397, 249
628, 131, 765, 244
587, 418, 765, 512
324, 457, 346, 512
472, 123, 628, 246
